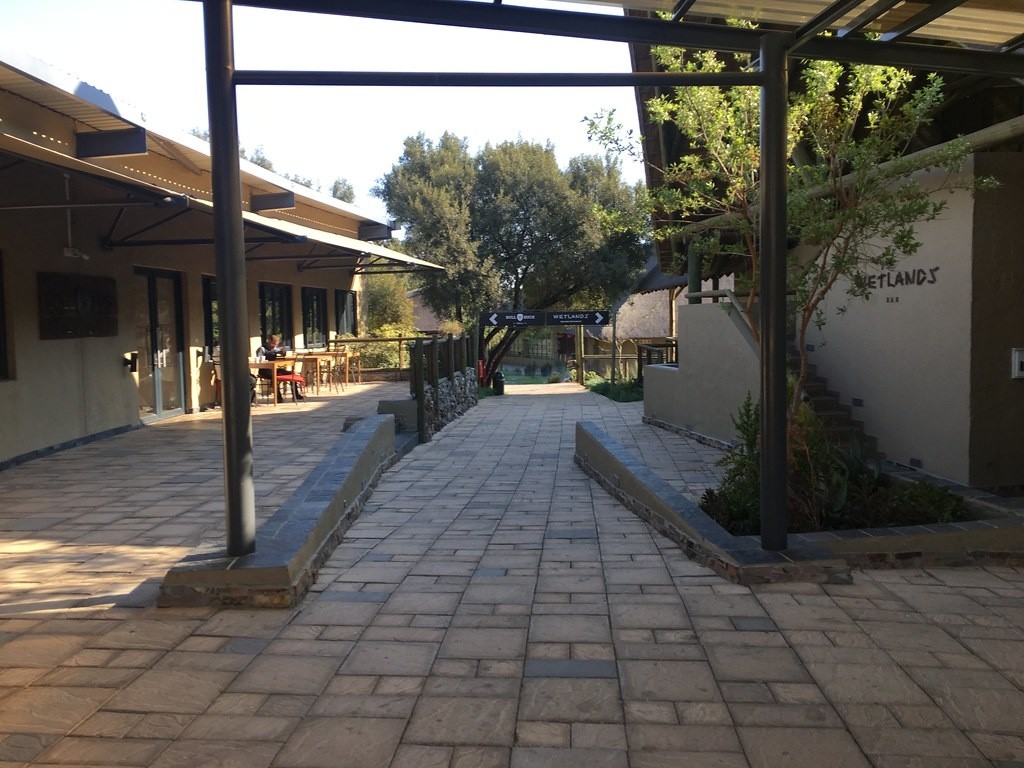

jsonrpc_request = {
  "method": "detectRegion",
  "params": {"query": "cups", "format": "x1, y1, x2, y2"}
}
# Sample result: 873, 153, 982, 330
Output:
259, 356, 265, 363
255, 357, 260, 363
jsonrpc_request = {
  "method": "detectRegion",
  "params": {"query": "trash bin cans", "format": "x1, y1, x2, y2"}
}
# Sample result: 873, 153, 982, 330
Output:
493, 371, 505, 395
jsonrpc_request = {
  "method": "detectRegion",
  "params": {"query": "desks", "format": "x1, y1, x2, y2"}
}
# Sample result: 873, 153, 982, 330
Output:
276, 356, 333, 395
293, 352, 361, 387
249, 361, 298, 408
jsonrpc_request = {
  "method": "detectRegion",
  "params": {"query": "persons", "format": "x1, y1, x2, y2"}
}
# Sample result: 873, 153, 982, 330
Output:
256, 334, 304, 402
211, 342, 257, 405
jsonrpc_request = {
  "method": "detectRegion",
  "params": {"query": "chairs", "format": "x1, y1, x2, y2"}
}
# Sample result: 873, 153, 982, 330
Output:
212, 344, 362, 410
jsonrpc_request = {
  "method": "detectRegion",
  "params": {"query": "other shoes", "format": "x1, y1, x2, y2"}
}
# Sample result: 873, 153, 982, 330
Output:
296, 394, 303, 399
278, 398, 284, 403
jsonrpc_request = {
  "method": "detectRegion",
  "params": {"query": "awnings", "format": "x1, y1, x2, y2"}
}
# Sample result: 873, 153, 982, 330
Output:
0, 130, 445, 276
